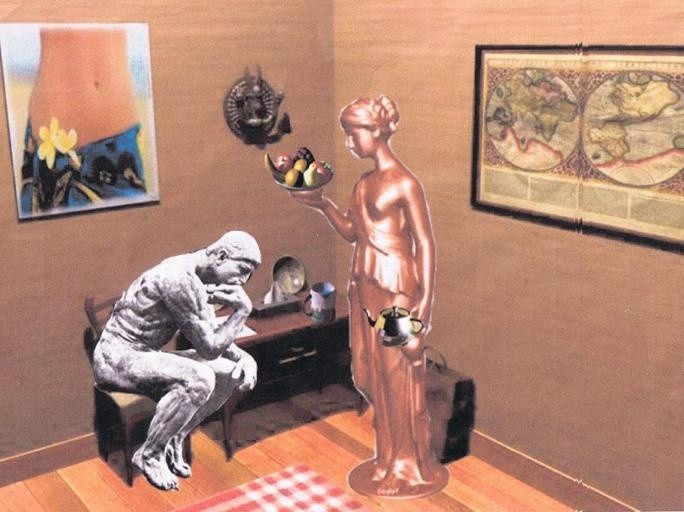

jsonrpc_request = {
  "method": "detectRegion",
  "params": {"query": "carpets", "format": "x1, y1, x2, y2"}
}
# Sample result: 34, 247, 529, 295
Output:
168, 463, 374, 512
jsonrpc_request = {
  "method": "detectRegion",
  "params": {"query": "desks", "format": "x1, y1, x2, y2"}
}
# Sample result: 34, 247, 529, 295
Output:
176, 288, 363, 462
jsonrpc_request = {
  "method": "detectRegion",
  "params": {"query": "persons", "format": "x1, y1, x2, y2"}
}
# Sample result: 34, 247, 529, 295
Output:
19, 26, 153, 211
287, 95, 436, 496
92, 229, 261, 494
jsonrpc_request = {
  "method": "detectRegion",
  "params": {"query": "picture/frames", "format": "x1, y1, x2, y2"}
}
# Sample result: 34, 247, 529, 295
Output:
469, 43, 684, 254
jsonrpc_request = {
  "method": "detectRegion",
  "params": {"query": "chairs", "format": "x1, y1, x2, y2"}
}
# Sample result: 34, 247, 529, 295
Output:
83, 295, 241, 487
